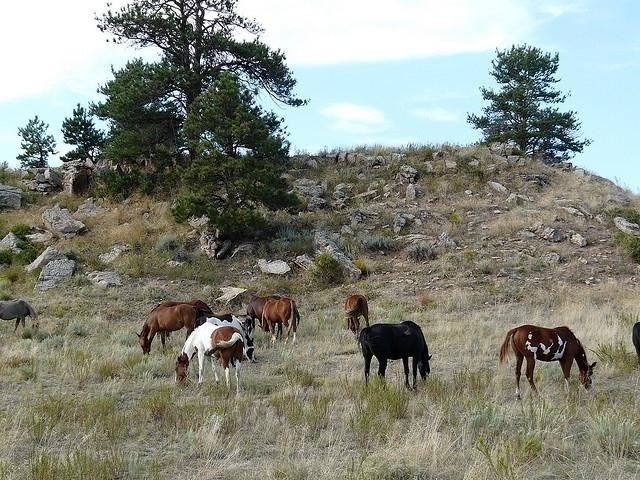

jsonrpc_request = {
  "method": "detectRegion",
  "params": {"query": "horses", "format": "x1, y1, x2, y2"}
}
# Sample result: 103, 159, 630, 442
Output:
135, 294, 299, 400
344, 295, 369, 340
0, 299, 37, 334
498, 324, 597, 401
357, 321, 433, 393
